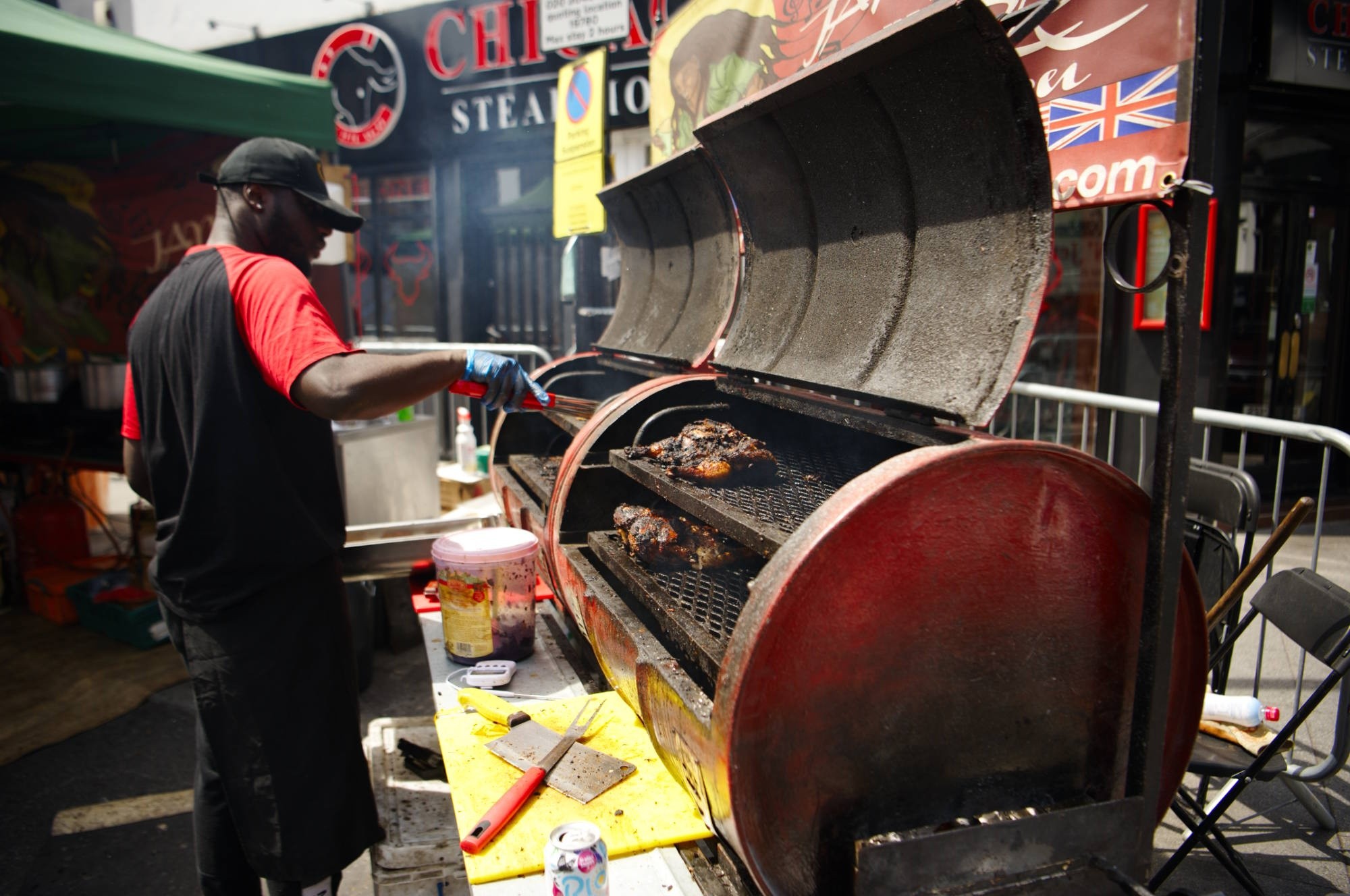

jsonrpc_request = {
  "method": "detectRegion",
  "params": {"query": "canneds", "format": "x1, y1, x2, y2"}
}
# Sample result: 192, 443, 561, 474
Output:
545, 821, 610, 896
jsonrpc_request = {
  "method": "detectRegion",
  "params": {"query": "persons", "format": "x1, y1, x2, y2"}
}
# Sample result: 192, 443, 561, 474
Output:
120, 136, 549, 896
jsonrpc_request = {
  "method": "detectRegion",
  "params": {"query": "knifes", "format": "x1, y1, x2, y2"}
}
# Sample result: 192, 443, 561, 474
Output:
460, 687, 636, 804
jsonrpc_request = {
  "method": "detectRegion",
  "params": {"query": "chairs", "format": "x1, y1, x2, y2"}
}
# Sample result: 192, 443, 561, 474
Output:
1140, 567, 1348, 894
1183, 456, 1260, 814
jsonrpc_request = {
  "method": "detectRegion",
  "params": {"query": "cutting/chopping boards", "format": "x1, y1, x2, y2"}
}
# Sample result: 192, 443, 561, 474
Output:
431, 688, 715, 885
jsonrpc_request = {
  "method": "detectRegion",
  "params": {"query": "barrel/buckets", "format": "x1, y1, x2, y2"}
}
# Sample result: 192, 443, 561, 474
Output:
432, 526, 540, 665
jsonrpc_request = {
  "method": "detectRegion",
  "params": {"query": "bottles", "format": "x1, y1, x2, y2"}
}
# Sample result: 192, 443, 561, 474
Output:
1201, 693, 1280, 727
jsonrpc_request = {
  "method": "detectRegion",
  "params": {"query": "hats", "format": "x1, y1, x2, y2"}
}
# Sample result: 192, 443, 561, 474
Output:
199, 136, 363, 232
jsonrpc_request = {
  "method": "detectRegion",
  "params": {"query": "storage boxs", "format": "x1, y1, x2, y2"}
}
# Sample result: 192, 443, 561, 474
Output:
360, 718, 463, 869
370, 840, 469, 896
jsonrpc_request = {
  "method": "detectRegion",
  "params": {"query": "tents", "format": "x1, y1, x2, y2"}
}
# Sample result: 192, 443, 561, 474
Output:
0, 0, 338, 151
